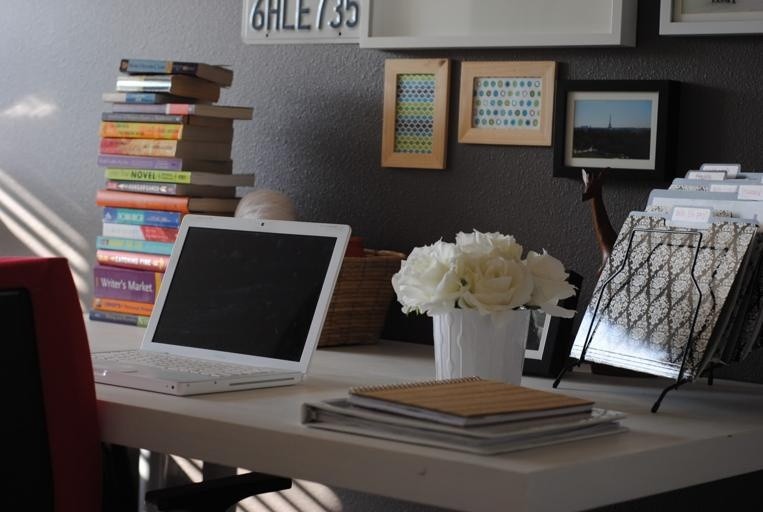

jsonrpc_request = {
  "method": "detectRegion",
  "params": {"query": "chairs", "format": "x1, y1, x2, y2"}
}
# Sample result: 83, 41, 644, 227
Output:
1, 251, 293, 509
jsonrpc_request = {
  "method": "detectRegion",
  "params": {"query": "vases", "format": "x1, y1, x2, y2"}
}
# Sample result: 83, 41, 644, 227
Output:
431, 309, 531, 385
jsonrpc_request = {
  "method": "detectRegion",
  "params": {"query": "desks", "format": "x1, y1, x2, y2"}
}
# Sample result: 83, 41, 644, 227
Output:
80, 311, 762, 512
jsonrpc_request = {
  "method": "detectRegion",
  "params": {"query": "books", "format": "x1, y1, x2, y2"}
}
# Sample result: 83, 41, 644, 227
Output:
87, 57, 257, 328
346, 373, 597, 429
298, 398, 631, 457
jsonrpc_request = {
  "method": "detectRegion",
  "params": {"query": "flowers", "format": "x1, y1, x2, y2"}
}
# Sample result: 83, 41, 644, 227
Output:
392, 227, 578, 322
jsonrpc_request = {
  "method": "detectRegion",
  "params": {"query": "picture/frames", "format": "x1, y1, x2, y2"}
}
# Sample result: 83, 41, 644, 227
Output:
553, 78, 675, 180
458, 60, 559, 147
656, 1, 760, 36
359, 2, 636, 48
380, 58, 453, 171
524, 270, 584, 374
359, 0, 762, 182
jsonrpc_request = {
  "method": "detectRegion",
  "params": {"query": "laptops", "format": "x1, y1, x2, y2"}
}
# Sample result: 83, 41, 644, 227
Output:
91, 214, 352, 395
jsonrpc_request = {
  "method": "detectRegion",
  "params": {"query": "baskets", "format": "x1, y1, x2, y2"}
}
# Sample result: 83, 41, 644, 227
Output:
317, 248, 406, 346
